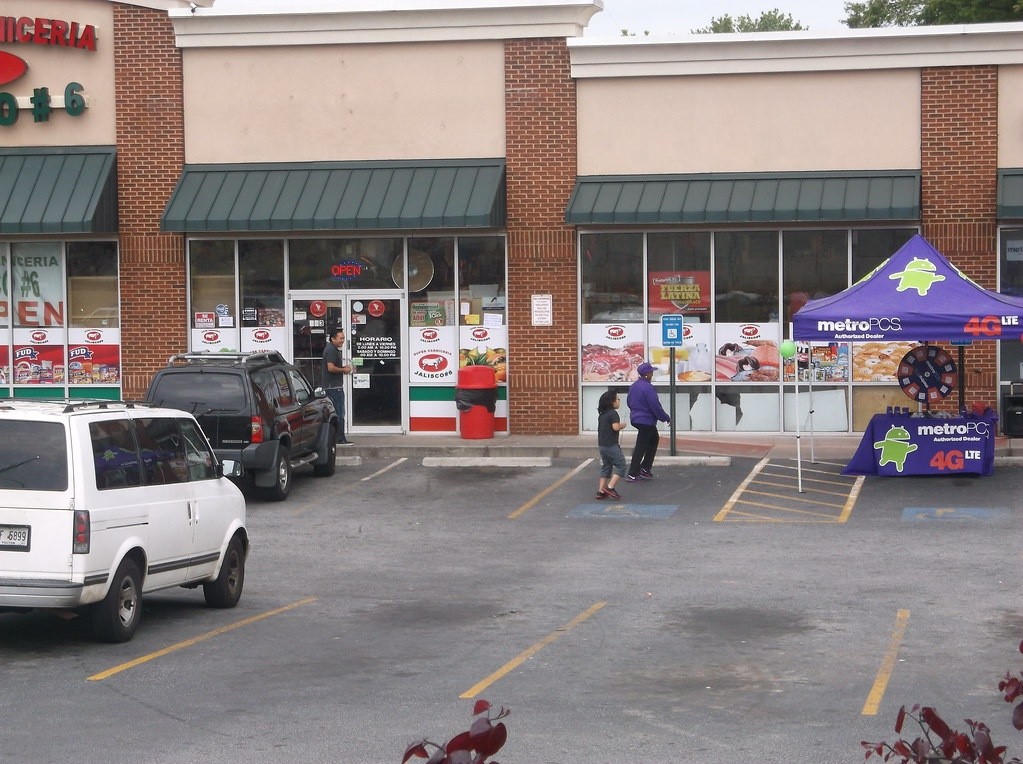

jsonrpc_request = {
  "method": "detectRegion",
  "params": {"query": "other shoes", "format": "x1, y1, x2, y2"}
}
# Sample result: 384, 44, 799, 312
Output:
336, 440, 354, 446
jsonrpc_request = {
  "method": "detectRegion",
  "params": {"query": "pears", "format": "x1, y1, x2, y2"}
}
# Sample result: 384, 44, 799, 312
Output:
461, 346, 506, 359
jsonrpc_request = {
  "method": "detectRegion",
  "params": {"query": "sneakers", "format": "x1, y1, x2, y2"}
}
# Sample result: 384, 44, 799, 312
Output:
596, 491, 609, 498
639, 469, 653, 479
625, 475, 647, 483
602, 484, 620, 499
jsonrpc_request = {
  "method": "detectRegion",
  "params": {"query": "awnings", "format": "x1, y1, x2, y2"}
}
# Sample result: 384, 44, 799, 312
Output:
160, 157, 507, 231
566, 170, 923, 226
996, 168, 1023, 219
0, 146, 117, 235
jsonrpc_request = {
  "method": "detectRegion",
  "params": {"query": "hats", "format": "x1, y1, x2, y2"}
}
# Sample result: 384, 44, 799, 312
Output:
637, 364, 658, 375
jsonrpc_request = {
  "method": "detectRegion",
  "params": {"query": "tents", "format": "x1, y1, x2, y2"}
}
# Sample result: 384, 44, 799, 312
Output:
792, 233, 1023, 492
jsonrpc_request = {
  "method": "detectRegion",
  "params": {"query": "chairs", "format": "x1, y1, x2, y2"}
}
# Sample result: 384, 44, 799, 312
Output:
199, 381, 224, 404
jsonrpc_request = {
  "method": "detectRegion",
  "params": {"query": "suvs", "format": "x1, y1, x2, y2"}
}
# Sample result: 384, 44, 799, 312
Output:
145, 350, 343, 503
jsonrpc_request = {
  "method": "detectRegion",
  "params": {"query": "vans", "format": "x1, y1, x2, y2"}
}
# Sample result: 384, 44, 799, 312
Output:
0, 396, 249, 642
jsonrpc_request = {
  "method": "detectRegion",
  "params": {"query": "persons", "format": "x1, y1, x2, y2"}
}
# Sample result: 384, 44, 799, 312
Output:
322, 330, 354, 445
595, 391, 626, 499
626, 363, 671, 482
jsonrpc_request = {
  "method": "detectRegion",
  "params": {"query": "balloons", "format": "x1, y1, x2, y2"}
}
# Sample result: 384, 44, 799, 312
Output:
780, 341, 796, 359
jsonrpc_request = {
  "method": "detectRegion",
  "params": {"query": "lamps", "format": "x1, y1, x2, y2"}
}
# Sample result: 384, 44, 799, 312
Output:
189, 3, 197, 14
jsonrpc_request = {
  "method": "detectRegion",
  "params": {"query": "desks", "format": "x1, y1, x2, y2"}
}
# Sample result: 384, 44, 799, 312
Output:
841, 408, 999, 478
